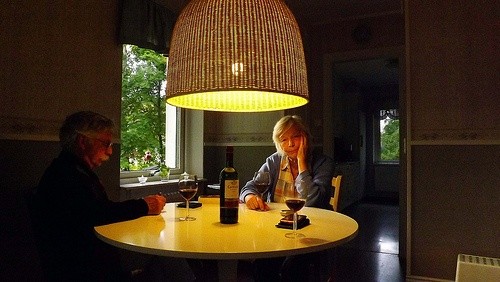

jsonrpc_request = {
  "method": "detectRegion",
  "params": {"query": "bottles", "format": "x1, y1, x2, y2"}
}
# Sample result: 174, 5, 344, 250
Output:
218, 146, 240, 224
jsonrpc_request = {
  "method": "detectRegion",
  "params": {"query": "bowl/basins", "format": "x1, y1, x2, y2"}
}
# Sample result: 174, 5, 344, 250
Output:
137, 176, 147, 183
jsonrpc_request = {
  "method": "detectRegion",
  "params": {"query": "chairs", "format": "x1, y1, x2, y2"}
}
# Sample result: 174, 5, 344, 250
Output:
326, 174, 343, 282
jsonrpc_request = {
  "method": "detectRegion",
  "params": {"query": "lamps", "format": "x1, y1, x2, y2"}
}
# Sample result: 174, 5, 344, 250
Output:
165, 0, 310, 112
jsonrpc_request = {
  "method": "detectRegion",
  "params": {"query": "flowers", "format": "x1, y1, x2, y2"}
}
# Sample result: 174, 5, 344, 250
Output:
142, 149, 169, 177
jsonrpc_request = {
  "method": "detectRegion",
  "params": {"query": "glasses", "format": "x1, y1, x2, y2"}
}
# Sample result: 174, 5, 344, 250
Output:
87, 134, 112, 148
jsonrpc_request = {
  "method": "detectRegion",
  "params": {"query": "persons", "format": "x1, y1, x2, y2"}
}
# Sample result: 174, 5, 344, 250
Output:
35, 111, 198, 282
240, 115, 337, 282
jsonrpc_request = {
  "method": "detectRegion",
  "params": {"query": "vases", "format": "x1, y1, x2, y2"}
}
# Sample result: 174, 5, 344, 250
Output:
161, 170, 170, 181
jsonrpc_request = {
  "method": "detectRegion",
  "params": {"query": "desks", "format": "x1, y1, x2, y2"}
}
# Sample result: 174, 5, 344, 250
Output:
94, 198, 359, 282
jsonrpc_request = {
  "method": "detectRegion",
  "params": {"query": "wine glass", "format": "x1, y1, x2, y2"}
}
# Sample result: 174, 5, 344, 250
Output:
176, 174, 198, 221
283, 180, 306, 239
